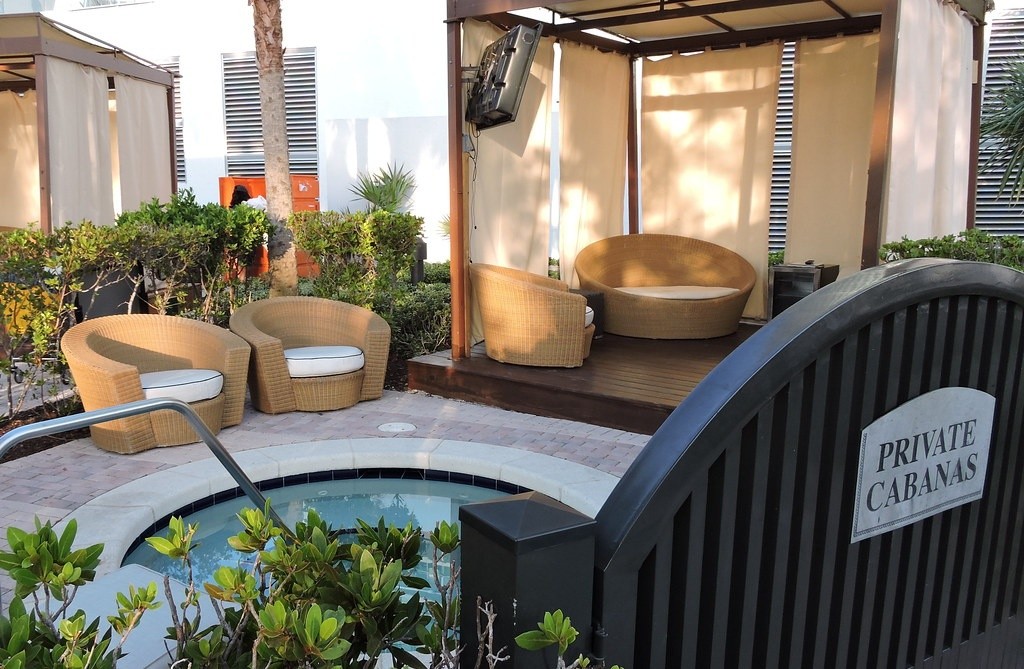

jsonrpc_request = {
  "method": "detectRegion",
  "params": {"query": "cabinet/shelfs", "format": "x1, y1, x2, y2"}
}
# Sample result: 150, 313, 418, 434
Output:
768, 264, 839, 320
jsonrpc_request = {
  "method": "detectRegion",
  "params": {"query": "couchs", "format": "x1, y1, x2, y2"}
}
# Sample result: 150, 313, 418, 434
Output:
60, 314, 251, 454
573, 232, 758, 340
229, 296, 391, 414
468, 264, 595, 367
